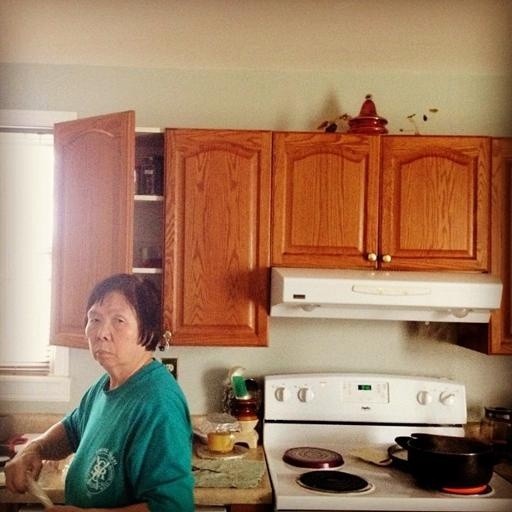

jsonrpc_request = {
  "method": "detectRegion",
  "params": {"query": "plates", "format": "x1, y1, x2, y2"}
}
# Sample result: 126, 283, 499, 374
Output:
196, 444, 249, 460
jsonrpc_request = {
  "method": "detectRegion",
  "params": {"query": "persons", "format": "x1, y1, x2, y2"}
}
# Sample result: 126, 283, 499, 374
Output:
4, 274, 195, 512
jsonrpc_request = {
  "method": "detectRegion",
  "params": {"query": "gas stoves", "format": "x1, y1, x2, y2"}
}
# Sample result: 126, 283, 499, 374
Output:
263, 443, 511, 498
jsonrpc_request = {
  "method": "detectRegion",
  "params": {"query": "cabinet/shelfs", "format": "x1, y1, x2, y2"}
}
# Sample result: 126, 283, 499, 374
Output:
48, 108, 272, 347
490, 136, 512, 356
271, 129, 490, 273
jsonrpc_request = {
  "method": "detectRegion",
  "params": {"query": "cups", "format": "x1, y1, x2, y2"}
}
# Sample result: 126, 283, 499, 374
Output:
206, 432, 235, 453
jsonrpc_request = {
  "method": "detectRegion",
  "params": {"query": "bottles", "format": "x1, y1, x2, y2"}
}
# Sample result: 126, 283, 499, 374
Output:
0, 456, 11, 487
141, 246, 153, 268
139, 155, 161, 195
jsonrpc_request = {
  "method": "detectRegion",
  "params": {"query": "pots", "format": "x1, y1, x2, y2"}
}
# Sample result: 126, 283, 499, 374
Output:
395, 434, 500, 488
